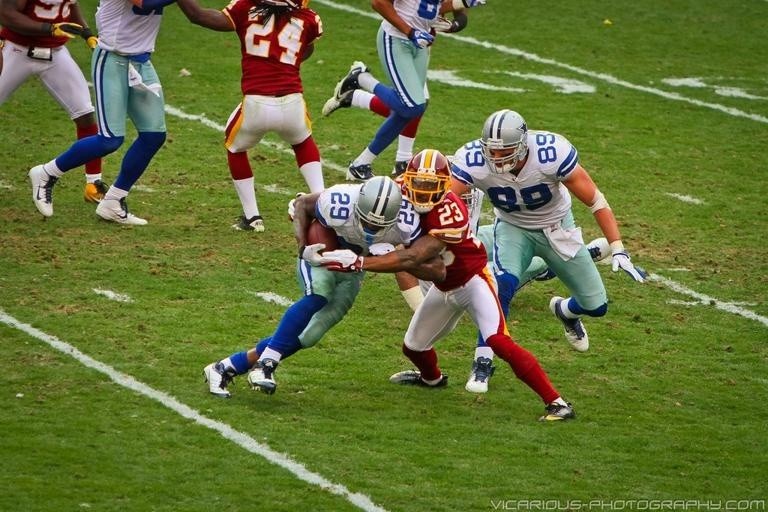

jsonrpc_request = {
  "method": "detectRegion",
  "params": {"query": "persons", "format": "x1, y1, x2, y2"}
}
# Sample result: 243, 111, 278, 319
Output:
0, 0, 110, 205
175, 0, 325, 234
287, 148, 576, 424
445, 108, 653, 395
201, 175, 447, 399
333, 0, 469, 184
28, 1, 178, 226
392, 185, 611, 314
321, 0, 487, 176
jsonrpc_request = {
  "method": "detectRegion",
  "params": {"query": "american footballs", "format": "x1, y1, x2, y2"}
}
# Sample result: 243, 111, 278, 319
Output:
306, 219, 337, 253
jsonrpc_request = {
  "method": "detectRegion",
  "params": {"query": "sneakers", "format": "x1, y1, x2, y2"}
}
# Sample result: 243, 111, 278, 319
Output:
346, 163, 375, 181
84, 180, 109, 203
466, 356, 496, 394
231, 215, 265, 232
247, 358, 278, 395
539, 401, 576, 422
203, 361, 237, 399
334, 61, 371, 102
28, 163, 59, 218
390, 369, 448, 387
391, 162, 408, 176
550, 296, 589, 352
584, 238, 612, 262
95, 196, 148, 227
321, 80, 356, 117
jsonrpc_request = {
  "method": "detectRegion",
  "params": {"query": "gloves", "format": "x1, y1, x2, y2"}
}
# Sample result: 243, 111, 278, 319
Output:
51, 22, 83, 39
322, 249, 364, 273
431, 16, 453, 32
408, 27, 435, 49
302, 243, 339, 267
611, 253, 648, 285
288, 192, 307, 222
369, 243, 396, 256
86, 35, 98, 50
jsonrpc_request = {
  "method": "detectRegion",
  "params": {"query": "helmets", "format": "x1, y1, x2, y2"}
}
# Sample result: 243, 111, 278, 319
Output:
481, 109, 528, 174
404, 149, 453, 214
353, 175, 402, 244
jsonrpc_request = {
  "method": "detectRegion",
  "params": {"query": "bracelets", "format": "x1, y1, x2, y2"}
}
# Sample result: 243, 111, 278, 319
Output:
588, 194, 606, 214
